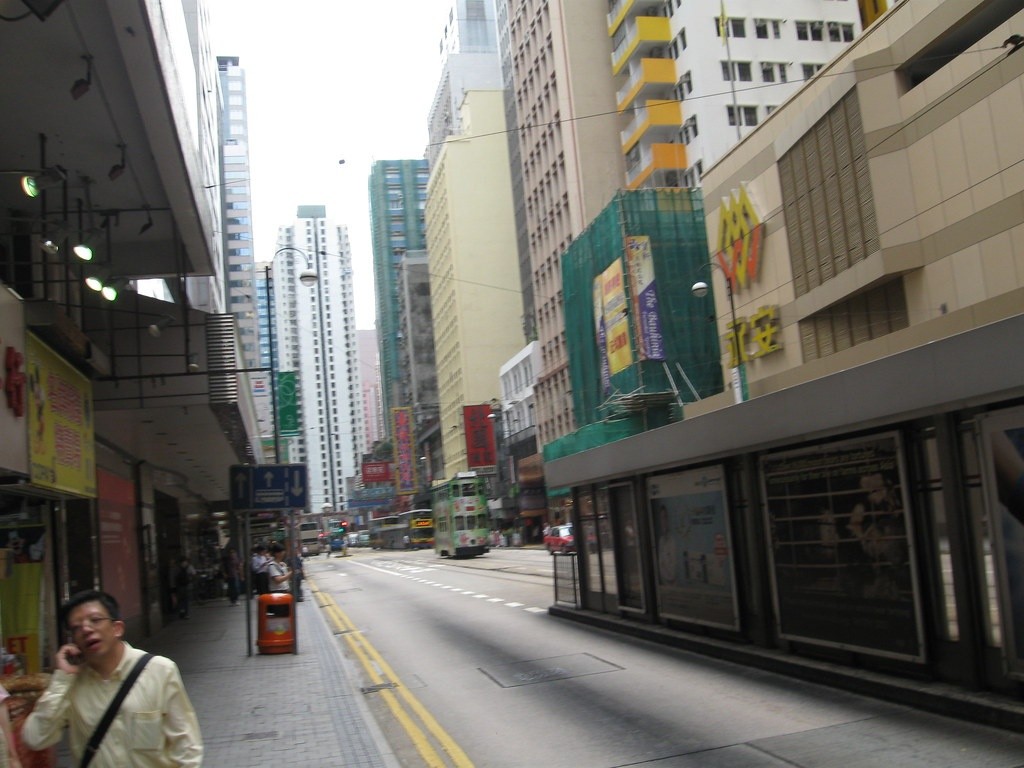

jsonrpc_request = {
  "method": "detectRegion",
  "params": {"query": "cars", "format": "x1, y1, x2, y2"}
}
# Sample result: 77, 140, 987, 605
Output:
543, 523, 597, 556
347, 528, 371, 548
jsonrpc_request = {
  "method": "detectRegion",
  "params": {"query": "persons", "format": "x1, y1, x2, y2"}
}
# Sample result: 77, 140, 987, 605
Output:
402, 533, 411, 549
658, 506, 679, 587
21, 590, 205, 768
0, 683, 22, 768
164, 540, 310, 626
321, 533, 351, 559
490, 522, 551, 549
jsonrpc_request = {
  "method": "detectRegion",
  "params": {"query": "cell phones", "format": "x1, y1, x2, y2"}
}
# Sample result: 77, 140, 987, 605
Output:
70, 653, 85, 664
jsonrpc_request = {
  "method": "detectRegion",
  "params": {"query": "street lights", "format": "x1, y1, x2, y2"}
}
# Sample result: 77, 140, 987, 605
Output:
693, 261, 747, 404
264, 246, 319, 464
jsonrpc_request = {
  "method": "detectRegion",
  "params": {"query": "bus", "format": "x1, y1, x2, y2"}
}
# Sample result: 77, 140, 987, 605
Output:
367, 508, 435, 551
297, 521, 319, 557
429, 471, 491, 560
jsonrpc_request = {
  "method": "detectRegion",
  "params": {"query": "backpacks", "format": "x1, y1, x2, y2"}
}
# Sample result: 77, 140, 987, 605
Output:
177, 565, 190, 585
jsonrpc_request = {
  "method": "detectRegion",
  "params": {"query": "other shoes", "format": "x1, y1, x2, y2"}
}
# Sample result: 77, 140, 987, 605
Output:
298, 597, 304, 603
236, 600, 240, 606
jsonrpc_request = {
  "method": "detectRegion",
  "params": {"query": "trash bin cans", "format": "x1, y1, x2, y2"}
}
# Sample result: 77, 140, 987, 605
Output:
256, 593, 295, 655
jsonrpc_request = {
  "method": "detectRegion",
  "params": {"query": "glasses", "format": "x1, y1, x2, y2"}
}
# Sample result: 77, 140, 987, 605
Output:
66, 618, 115, 638
